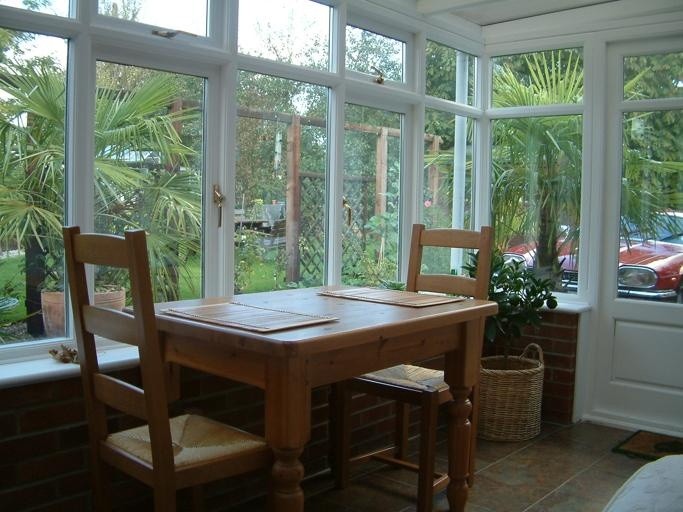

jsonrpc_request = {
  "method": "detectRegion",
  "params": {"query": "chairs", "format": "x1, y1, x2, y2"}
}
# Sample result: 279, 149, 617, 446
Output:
62, 226, 270, 511
332, 223, 494, 510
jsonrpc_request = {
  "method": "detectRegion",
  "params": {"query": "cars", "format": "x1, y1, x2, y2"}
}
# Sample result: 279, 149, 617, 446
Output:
501, 209, 682, 304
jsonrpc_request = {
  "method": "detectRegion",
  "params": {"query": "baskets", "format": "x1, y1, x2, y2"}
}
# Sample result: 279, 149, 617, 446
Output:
472, 342, 548, 444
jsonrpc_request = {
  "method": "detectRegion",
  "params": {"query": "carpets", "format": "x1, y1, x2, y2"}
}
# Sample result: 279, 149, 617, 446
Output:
613, 426, 681, 465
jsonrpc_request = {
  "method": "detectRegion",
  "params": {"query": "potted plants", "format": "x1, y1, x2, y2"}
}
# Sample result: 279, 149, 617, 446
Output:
1, 44, 210, 331
449, 241, 575, 447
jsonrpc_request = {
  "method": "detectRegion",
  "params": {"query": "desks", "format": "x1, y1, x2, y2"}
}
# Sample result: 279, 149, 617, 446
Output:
121, 284, 497, 510
